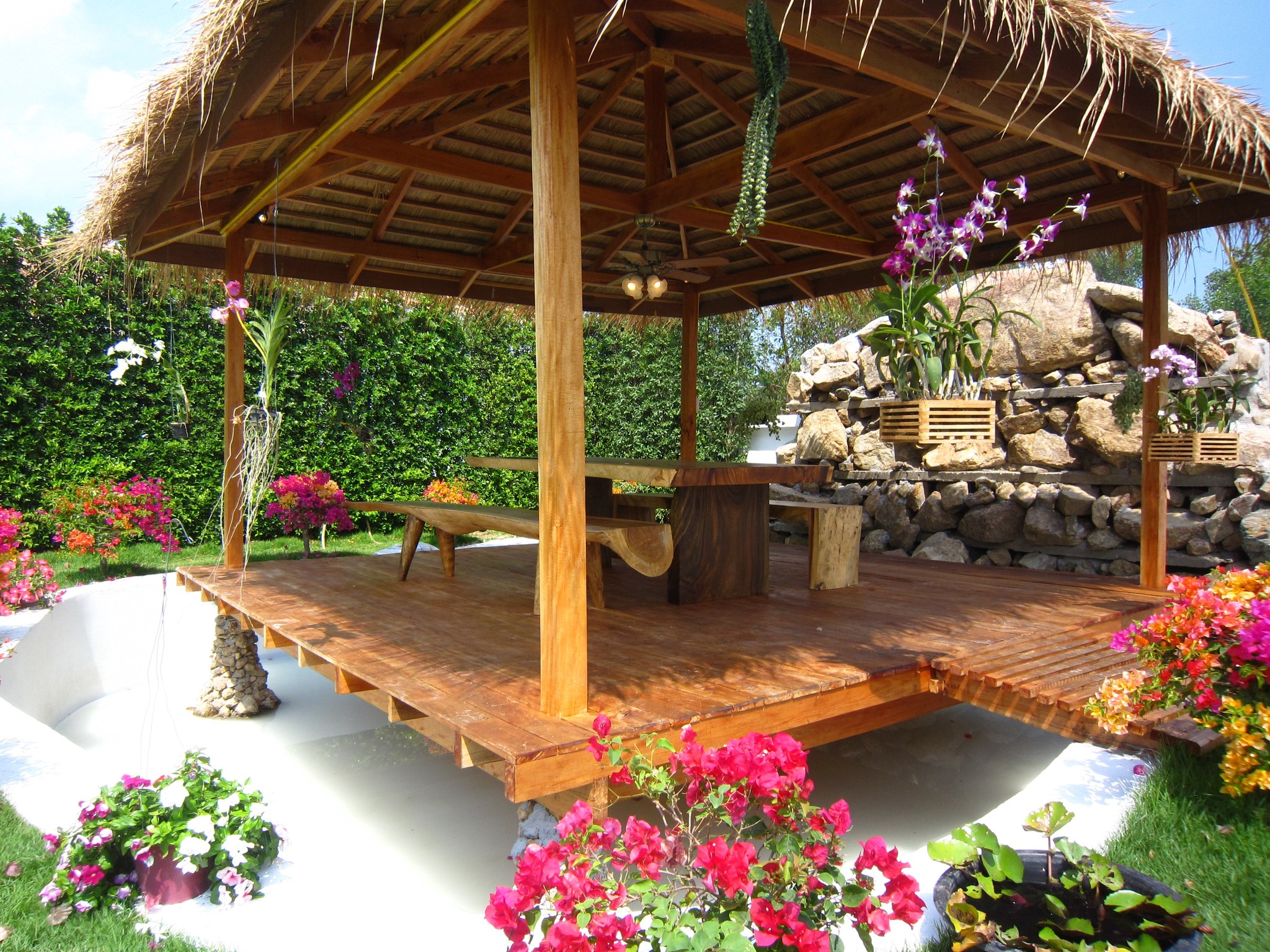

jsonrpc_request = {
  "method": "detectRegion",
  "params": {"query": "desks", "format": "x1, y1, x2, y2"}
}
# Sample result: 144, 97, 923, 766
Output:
467, 456, 833, 604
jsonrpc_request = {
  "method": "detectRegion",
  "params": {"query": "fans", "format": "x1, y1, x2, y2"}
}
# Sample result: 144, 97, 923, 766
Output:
604, 214, 731, 286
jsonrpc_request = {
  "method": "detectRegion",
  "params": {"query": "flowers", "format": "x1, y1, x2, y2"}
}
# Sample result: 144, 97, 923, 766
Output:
484, 713, 926, 952
108, 337, 190, 423
196, 279, 297, 599
334, 361, 365, 432
866, 126, 1091, 400
39, 747, 286, 912
1107, 342, 1261, 434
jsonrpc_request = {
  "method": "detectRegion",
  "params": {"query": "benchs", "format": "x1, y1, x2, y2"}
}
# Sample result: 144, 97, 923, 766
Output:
613, 493, 862, 590
346, 501, 675, 615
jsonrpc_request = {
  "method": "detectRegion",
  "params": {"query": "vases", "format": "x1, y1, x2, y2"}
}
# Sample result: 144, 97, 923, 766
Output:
133, 846, 211, 904
879, 398, 995, 444
172, 423, 193, 440
246, 408, 276, 430
1148, 430, 1239, 463
359, 433, 369, 442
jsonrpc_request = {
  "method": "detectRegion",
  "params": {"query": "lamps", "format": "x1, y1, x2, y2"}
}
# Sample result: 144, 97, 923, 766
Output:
621, 274, 668, 300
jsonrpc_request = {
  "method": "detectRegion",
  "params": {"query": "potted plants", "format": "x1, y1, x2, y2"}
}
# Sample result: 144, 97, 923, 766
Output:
724, 350, 796, 442
926, 803, 1208, 952
